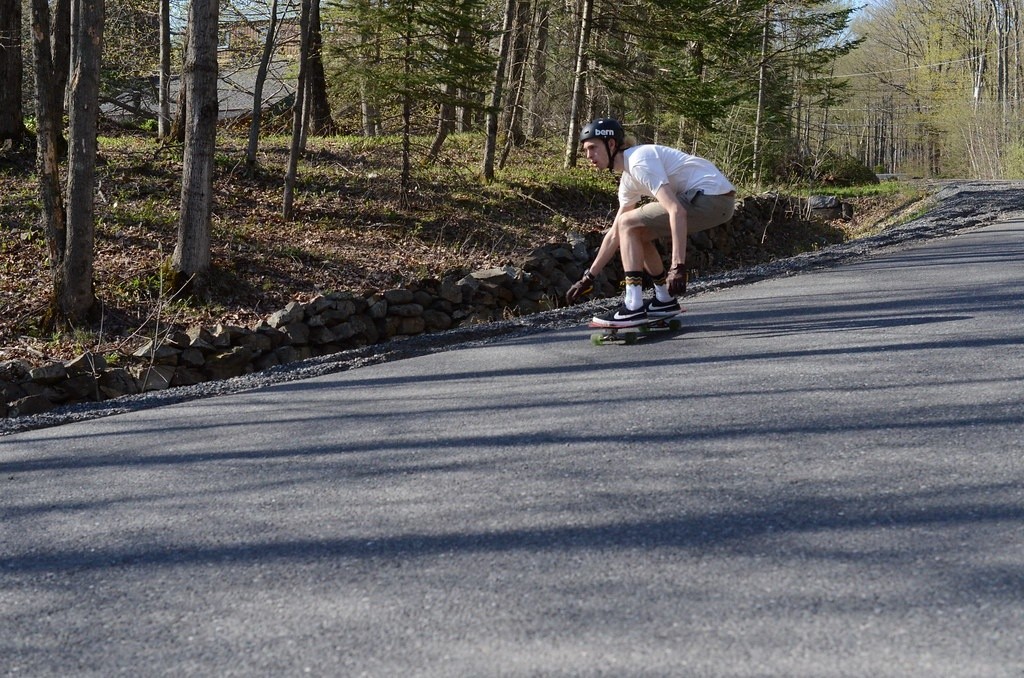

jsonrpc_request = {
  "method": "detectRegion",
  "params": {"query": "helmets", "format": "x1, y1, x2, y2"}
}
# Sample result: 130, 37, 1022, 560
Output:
579, 118, 625, 144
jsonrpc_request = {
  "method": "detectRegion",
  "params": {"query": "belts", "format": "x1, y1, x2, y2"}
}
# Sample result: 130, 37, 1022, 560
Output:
722, 190, 736, 197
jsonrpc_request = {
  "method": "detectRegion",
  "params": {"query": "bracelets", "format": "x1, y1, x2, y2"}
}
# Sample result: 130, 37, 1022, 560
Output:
584, 268, 594, 283
670, 263, 686, 270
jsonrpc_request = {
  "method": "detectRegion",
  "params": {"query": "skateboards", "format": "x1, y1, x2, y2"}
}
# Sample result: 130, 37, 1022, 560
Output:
588, 304, 687, 345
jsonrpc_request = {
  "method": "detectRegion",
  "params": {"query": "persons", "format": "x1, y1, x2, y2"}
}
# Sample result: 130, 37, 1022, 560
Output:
565, 117, 738, 326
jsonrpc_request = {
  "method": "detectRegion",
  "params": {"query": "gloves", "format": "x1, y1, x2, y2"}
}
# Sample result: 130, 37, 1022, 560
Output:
666, 263, 689, 297
566, 275, 593, 306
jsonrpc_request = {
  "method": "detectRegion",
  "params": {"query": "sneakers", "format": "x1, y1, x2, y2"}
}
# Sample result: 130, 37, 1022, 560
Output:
644, 296, 681, 316
593, 302, 649, 325
